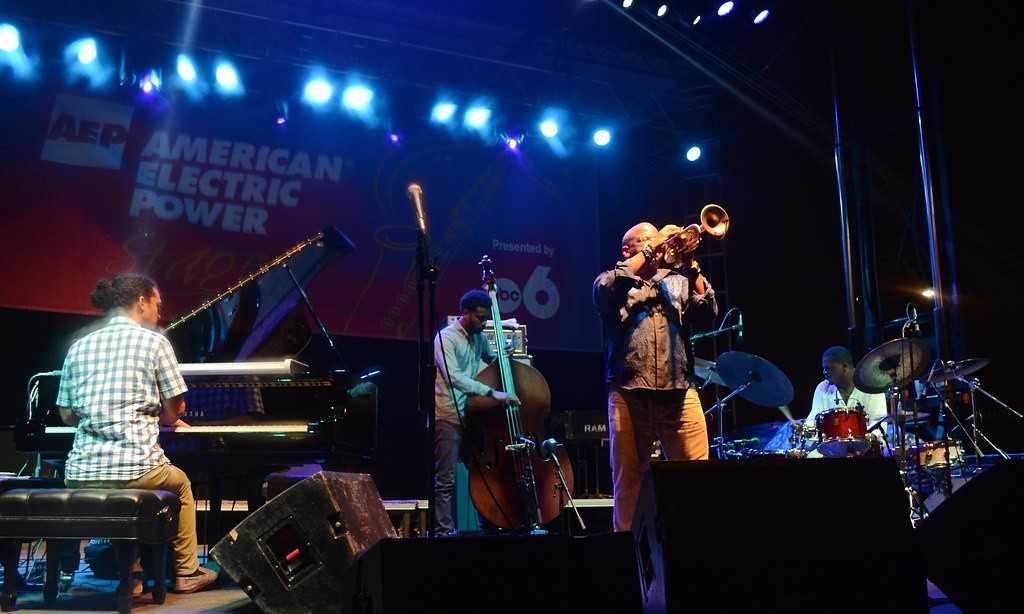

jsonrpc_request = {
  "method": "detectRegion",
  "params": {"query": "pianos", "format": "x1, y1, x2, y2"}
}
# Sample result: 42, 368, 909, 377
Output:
13, 224, 377, 566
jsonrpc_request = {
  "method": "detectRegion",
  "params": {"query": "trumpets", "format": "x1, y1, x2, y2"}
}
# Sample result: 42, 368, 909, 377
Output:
652, 203, 730, 261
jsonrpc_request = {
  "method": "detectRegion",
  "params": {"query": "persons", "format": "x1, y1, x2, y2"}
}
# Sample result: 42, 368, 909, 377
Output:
792, 346, 887, 457
433, 285, 521, 538
53, 271, 220, 592
593, 222, 719, 532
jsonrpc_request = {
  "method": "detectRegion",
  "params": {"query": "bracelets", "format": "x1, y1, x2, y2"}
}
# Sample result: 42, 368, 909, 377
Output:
641, 244, 656, 262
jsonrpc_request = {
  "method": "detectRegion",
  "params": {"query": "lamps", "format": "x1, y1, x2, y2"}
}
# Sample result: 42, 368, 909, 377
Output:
496, 114, 527, 149
263, 85, 289, 128
117, 43, 167, 94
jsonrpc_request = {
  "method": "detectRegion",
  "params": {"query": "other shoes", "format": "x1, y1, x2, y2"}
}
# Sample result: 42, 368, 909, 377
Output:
433, 527, 461, 537
174, 560, 222, 592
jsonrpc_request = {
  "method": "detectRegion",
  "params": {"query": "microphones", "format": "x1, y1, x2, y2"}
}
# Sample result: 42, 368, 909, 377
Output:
948, 412, 979, 438
738, 313, 744, 344
867, 416, 888, 433
408, 185, 426, 235
722, 219, 729, 223
543, 439, 560, 471
836, 395, 839, 405
919, 364, 937, 407
515, 434, 536, 445
912, 305, 922, 337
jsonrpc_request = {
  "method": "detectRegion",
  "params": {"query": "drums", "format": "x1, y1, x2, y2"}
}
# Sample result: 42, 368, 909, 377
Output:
907, 440, 966, 469
814, 406, 870, 454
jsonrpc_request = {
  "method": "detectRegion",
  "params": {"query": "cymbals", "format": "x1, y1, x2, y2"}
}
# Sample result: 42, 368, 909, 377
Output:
715, 351, 794, 406
918, 356, 992, 385
875, 411, 931, 424
852, 336, 934, 394
692, 357, 727, 386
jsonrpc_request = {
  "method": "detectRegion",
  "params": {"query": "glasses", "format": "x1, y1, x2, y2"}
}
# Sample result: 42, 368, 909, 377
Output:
136, 293, 164, 315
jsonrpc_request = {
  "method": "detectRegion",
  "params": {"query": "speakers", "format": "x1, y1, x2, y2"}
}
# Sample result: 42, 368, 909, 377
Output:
208, 470, 399, 614
565, 442, 615, 500
340, 529, 644, 614
630, 455, 929, 614
911, 456, 1024, 614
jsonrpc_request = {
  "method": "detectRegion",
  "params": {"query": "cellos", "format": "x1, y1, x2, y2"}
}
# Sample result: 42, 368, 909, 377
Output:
461, 254, 574, 533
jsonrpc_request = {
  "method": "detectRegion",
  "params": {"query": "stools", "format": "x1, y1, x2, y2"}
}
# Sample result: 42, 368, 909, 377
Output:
0, 488, 183, 614
